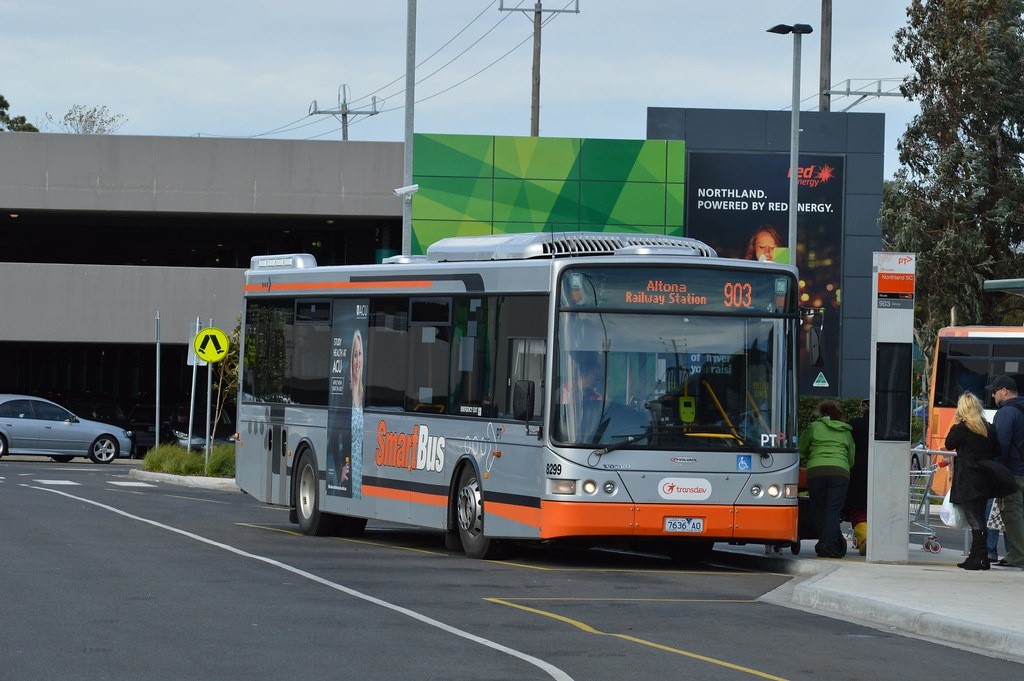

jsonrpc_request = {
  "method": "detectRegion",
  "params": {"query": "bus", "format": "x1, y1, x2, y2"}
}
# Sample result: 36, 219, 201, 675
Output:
225, 231, 827, 564
923, 323, 1024, 499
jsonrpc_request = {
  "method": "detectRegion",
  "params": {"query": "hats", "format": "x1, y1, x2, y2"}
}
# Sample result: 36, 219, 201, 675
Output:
984, 374, 1017, 391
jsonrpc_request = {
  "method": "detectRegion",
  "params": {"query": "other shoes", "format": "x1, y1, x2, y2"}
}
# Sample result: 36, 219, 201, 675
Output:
991, 558, 1022, 570
816, 542, 841, 558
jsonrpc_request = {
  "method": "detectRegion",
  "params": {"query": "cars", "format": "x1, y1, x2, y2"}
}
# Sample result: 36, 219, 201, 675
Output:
1, 391, 136, 465
911, 443, 926, 480
126, 402, 175, 462
169, 402, 237, 456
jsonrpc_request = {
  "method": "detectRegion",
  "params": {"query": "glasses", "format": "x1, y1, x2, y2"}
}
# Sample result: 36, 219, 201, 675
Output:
993, 390, 997, 395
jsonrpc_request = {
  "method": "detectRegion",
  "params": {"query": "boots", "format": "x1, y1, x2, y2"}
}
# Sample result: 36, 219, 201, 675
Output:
957, 529, 990, 570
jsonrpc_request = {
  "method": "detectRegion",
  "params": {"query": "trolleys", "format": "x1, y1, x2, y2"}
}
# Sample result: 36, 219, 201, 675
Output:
909, 449, 958, 554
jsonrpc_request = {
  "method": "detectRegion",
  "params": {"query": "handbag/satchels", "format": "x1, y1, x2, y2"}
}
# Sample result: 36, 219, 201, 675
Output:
939, 487, 960, 528
931, 447, 956, 495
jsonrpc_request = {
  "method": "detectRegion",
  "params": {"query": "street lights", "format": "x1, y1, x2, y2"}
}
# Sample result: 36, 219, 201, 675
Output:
766, 23, 814, 267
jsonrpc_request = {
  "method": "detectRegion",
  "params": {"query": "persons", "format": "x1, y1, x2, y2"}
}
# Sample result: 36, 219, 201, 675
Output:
332, 330, 364, 501
798, 399, 869, 558
743, 225, 787, 262
945, 375, 1024, 570
560, 370, 603, 404
